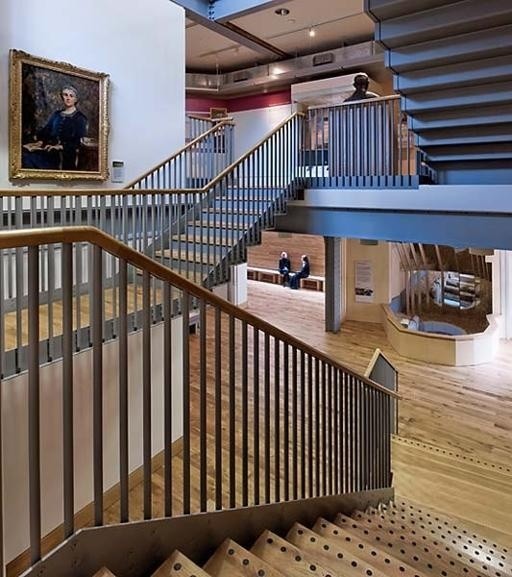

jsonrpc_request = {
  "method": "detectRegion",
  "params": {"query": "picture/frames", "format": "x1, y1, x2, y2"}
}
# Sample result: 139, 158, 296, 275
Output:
9, 48, 112, 185
210, 107, 228, 119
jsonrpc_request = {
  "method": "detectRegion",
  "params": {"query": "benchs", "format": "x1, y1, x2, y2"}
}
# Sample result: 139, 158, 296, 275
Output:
248, 267, 325, 293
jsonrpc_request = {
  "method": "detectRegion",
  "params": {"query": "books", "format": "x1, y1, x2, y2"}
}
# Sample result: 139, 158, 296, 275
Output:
23, 145, 44, 151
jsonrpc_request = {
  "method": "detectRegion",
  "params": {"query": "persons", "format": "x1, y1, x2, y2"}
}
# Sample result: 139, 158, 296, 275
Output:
343, 73, 380, 102
290, 255, 309, 289
279, 252, 290, 287
24, 85, 88, 169
